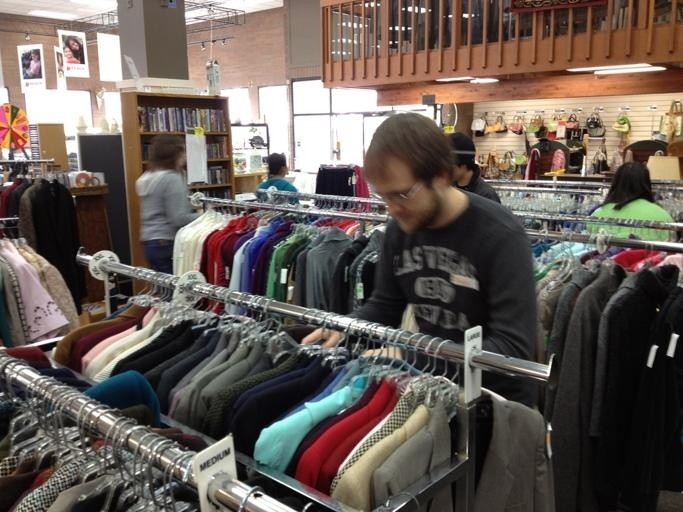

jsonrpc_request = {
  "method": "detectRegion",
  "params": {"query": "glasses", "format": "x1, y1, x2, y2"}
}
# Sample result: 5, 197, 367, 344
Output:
371, 182, 421, 206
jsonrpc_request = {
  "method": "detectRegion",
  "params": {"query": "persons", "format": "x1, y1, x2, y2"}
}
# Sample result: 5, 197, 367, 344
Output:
299, 112, 538, 410
137, 106, 233, 214
22, 51, 41, 79
585, 161, 677, 243
134, 131, 208, 302
64, 37, 84, 64
443, 132, 501, 204
62, 47, 80, 64
253, 151, 300, 207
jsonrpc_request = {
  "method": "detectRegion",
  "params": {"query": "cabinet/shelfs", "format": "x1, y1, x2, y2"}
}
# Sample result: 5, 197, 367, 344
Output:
120, 92, 235, 294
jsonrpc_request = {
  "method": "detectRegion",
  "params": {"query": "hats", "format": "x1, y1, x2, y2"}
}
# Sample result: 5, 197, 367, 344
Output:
446, 131, 476, 166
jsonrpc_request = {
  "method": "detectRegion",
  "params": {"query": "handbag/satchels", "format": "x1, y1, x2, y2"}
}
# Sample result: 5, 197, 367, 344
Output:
471, 111, 631, 141
660, 101, 683, 137
478, 151, 529, 179
592, 144, 610, 174
